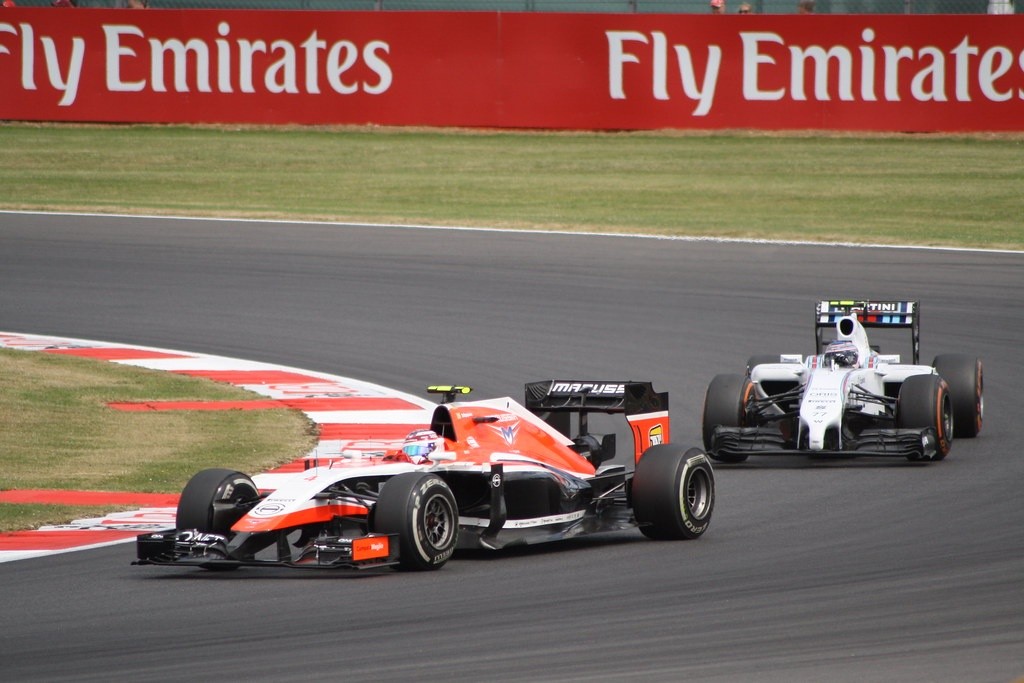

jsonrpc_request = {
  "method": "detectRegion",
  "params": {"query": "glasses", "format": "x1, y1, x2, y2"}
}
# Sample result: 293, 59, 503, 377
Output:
402, 445, 430, 457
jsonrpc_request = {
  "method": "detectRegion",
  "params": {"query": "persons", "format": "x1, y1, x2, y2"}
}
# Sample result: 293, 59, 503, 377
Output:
128, 0, 150, 9
51, 0, 78, 8
739, 2, 752, 15
799, 0, 816, 15
710, 0, 727, 14
1, 0, 16, 7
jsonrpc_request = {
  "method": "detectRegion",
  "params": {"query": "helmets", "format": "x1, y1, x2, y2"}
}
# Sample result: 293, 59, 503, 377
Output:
401, 429, 438, 465
824, 340, 859, 366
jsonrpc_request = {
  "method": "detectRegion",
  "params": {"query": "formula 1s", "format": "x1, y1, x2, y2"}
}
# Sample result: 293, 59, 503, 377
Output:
702, 296, 986, 466
134, 377, 718, 582
